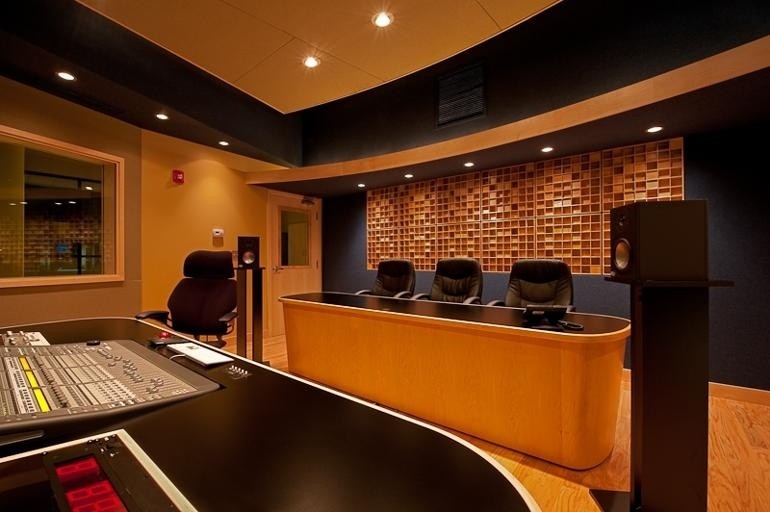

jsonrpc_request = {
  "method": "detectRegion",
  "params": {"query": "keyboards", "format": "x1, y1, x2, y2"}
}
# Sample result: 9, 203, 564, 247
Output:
166, 340, 236, 369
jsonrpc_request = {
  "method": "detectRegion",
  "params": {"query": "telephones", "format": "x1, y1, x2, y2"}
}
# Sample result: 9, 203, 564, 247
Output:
520, 305, 567, 329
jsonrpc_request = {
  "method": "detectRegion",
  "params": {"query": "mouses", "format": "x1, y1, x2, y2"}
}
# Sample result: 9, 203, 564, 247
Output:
156, 330, 170, 339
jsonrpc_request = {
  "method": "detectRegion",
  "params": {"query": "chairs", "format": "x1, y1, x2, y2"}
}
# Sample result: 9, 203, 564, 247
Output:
355, 258, 576, 313
134, 250, 237, 348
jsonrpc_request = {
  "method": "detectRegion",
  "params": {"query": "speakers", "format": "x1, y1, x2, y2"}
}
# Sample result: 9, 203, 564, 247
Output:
238, 236, 261, 268
611, 199, 709, 284
